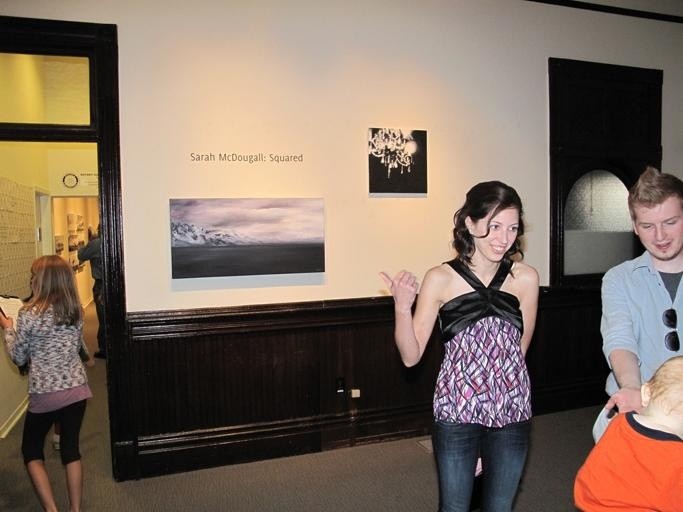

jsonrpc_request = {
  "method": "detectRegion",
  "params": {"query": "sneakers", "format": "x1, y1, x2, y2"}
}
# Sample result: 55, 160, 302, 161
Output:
94, 349, 107, 360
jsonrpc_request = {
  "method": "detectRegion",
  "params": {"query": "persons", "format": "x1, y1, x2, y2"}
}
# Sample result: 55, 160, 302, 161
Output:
600, 165, 683, 416
0, 255, 93, 512
377, 180, 540, 512
574, 353, 683, 512
15, 276, 94, 451
77, 221, 105, 359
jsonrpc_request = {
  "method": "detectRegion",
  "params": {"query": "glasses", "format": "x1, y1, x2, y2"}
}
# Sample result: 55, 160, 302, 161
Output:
662, 309, 680, 353
